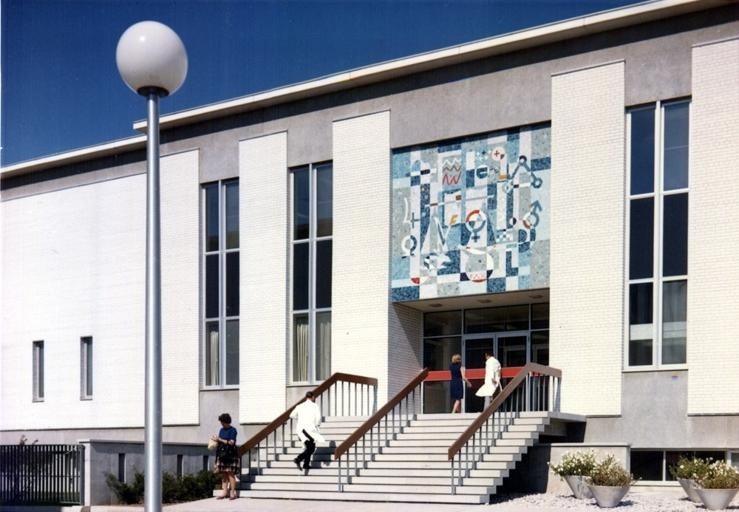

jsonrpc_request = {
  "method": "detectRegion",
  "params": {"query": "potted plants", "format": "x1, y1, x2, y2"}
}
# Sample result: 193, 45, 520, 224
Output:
547, 449, 633, 507
668, 451, 739, 510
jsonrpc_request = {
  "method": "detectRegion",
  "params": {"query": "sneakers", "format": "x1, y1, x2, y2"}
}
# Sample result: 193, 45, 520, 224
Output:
303, 464, 313, 469
294, 458, 302, 471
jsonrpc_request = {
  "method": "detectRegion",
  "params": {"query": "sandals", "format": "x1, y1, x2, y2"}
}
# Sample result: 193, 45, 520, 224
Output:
229, 488, 237, 500
216, 489, 229, 500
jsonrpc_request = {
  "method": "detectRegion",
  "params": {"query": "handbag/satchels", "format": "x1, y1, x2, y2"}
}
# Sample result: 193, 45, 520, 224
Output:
231, 441, 242, 459
218, 439, 234, 465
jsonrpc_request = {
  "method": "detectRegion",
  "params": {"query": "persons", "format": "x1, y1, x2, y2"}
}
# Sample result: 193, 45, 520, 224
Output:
447, 353, 474, 413
211, 411, 237, 501
289, 390, 322, 471
483, 348, 503, 412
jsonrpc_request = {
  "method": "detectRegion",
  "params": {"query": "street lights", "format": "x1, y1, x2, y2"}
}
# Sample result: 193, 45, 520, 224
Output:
110, 13, 194, 512
